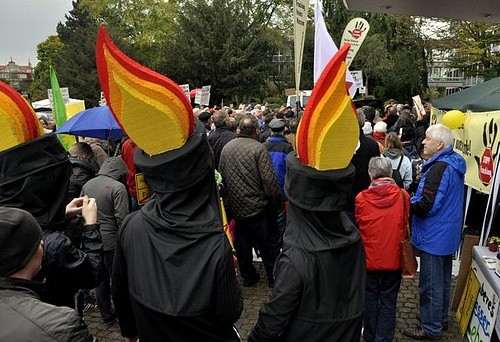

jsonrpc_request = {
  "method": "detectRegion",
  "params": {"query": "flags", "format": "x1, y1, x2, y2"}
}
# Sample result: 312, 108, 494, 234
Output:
49, 66, 68, 152
313, 3, 357, 100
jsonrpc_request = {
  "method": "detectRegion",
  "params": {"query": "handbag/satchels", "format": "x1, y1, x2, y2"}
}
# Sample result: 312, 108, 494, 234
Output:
401, 240, 418, 275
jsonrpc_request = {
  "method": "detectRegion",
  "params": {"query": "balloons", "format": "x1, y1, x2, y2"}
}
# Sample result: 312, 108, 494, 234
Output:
441, 110, 464, 129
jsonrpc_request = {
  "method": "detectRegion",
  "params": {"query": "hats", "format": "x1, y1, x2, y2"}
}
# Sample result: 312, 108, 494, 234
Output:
402, 106, 412, 111
279, 106, 286, 112
388, 99, 395, 103
289, 119, 299, 131
362, 122, 372, 134
269, 118, 285, 128
374, 121, 387, 133
198, 112, 213, 121
0, 207, 43, 276
254, 104, 261, 110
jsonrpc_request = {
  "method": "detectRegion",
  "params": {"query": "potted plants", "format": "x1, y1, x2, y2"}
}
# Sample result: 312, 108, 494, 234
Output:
487, 236, 500, 252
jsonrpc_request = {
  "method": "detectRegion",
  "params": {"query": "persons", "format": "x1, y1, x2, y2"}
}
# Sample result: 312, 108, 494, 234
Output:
247, 150, 366, 342
0, 116, 143, 319
402, 125, 466, 341
193, 95, 431, 240
112, 117, 244, 342
0, 207, 98, 342
354, 156, 418, 342
219, 115, 281, 288
76, 155, 129, 325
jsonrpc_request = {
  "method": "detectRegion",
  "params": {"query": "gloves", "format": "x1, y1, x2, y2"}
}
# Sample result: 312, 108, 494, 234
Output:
276, 210, 287, 236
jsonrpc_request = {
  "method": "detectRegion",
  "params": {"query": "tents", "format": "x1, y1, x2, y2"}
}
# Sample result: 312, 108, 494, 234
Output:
430, 76, 500, 245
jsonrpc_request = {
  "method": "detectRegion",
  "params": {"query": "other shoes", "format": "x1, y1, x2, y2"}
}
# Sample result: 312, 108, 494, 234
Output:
416, 315, 448, 331
104, 310, 116, 325
269, 279, 274, 287
403, 327, 441, 340
243, 272, 260, 287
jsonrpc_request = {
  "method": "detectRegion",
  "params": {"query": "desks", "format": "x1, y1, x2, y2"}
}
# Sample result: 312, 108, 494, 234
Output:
454, 246, 500, 342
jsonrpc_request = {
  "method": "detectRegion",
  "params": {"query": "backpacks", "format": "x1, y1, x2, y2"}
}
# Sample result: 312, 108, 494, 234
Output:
382, 154, 404, 189
402, 145, 424, 181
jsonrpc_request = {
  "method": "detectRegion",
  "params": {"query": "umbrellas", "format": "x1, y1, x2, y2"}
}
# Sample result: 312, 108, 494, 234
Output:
56, 106, 126, 142
